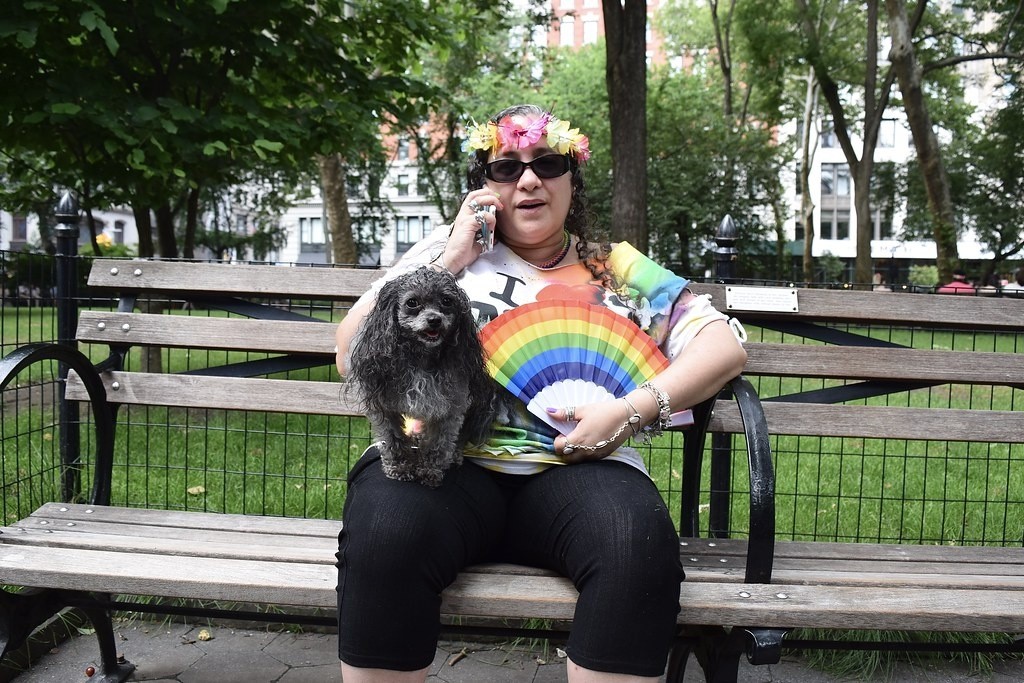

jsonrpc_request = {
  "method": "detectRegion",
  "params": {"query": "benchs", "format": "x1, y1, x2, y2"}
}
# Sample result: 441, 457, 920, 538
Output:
0, 258, 1024, 683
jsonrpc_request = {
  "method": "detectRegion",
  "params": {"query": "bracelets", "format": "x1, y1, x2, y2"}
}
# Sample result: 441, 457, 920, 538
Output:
429, 252, 456, 279
564, 381, 672, 455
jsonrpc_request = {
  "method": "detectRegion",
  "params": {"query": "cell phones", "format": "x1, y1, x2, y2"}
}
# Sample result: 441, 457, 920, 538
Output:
482, 204, 496, 252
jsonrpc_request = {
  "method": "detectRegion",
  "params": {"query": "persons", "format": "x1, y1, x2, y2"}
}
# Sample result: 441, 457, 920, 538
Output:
335, 102, 751, 683
936, 267, 1024, 298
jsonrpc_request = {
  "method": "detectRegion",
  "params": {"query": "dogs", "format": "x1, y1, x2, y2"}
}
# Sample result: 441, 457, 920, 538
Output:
339, 266, 522, 489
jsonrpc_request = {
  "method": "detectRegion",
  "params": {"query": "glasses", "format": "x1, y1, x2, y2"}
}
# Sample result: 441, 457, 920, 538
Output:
482, 153, 571, 183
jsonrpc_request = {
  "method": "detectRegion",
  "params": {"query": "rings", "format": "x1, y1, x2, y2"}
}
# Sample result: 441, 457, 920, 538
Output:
477, 237, 487, 253
468, 200, 483, 212
474, 210, 486, 225
565, 406, 576, 422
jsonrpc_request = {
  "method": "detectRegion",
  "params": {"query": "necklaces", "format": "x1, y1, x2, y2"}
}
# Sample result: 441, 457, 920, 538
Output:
535, 229, 572, 268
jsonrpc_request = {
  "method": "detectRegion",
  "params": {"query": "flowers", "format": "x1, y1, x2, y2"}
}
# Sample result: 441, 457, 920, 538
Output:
460, 111, 591, 164
